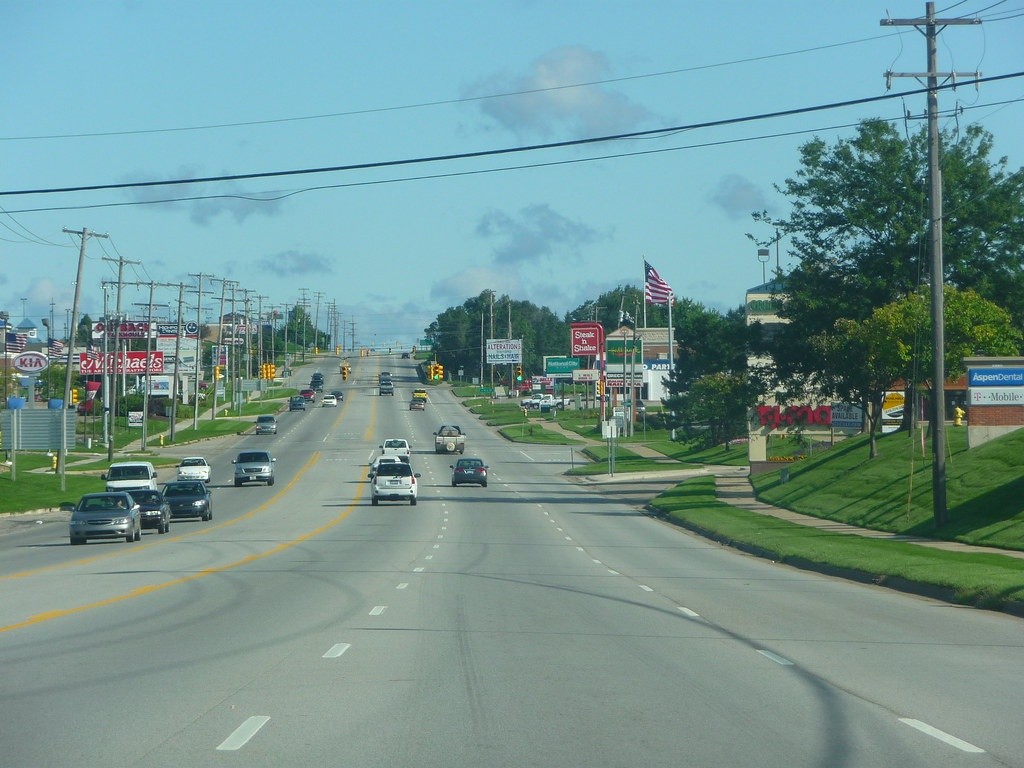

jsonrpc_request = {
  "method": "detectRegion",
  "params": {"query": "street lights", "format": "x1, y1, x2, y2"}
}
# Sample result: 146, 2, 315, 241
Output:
758, 245, 769, 288
40, 317, 52, 402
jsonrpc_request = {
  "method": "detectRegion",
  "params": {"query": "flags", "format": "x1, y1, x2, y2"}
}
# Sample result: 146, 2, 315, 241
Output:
5, 331, 28, 353
644, 258, 674, 308
48, 337, 65, 356
86, 348, 98, 360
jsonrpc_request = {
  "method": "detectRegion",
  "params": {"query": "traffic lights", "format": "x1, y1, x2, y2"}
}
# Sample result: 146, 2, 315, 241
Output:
342, 367, 347, 380
432, 365, 440, 380
72, 389, 78, 405
426, 366, 433, 379
270, 364, 276, 379
517, 365, 522, 380
259, 364, 266, 380
388, 348, 392, 354
437, 365, 444, 380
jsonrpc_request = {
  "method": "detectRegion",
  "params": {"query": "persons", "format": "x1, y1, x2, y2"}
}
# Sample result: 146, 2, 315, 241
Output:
189, 485, 203, 495
109, 499, 127, 510
386, 442, 406, 447
142, 494, 156, 504
137, 467, 146, 476
197, 460, 205, 466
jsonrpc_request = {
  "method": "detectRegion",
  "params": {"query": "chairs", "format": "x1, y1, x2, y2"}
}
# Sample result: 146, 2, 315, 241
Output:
88, 504, 102, 509
460, 462, 465, 466
474, 463, 479, 466
398, 443, 404, 446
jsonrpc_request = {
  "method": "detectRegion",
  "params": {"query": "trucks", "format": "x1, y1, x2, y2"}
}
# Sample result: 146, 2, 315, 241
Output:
433, 425, 467, 454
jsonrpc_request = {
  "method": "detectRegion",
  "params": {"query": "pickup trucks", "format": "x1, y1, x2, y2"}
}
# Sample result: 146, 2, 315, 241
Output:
520, 393, 570, 408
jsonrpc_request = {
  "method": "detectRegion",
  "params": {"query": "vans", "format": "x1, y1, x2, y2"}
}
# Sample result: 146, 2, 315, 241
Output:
101, 461, 158, 502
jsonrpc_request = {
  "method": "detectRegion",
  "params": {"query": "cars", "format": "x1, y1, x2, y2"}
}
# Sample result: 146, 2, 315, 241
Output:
230, 450, 276, 487
409, 389, 428, 410
173, 456, 212, 485
287, 372, 343, 412
367, 439, 422, 505
449, 457, 490, 488
70, 492, 141, 543
124, 489, 171, 535
253, 414, 278, 435
337, 362, 351, 375
157, 481, 213, 522
379, 370, 395, 396
401, 352, 411, 359
621, 398, 646, 413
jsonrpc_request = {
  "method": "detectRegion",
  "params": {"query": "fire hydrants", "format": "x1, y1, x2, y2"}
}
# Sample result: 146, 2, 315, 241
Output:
50, 456, 58, 472
224, 408, 229, 416
523, 409, 528, 417
953, 406, 966, 428
159, 434, 164, 445
